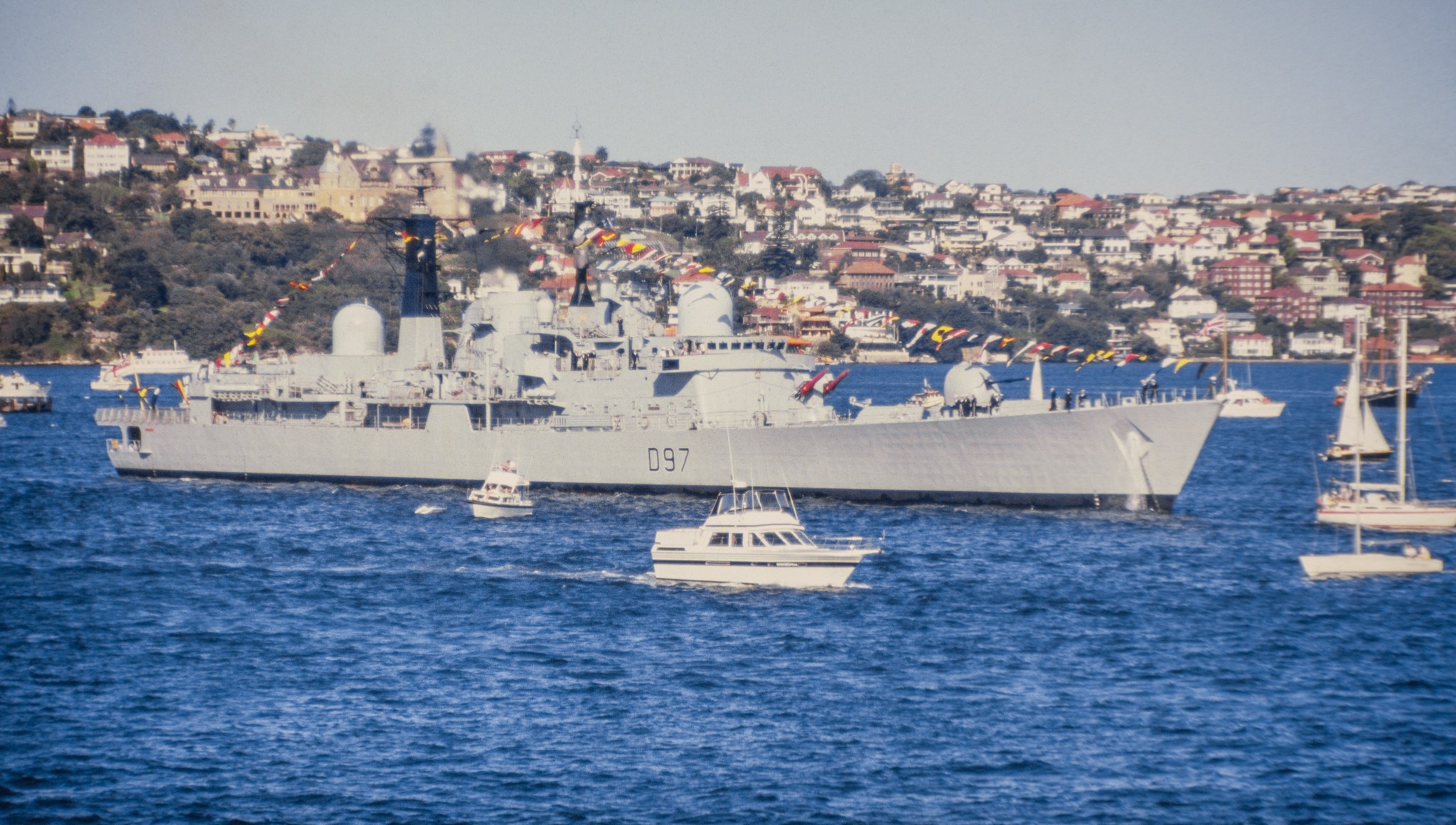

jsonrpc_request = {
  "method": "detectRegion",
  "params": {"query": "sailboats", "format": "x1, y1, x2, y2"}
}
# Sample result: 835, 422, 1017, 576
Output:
1296, 309, 1456, 582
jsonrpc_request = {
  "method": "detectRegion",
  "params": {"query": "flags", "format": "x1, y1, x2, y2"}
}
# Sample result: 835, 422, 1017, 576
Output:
393, 216, 1195, 374
176, 240, 356, 405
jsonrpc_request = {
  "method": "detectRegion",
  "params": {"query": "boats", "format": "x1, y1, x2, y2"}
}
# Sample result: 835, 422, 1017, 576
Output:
0, 370, 54, 413
1211, 304, 1286, 418
91, 338, 207, 392
647, 423, 887, 590
465, 442, 534, 518
90, 115, 1235, 518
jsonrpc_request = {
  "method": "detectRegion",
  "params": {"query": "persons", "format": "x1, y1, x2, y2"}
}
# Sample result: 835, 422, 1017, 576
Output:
1064, 388, 1073, 409
959, 395, 978, 417
990, 393, 999, 408
146, 385, 163, 411
1078, 389, 1087, 407
1049, 388, 1056, 411
1141, 379, 1160, 404
